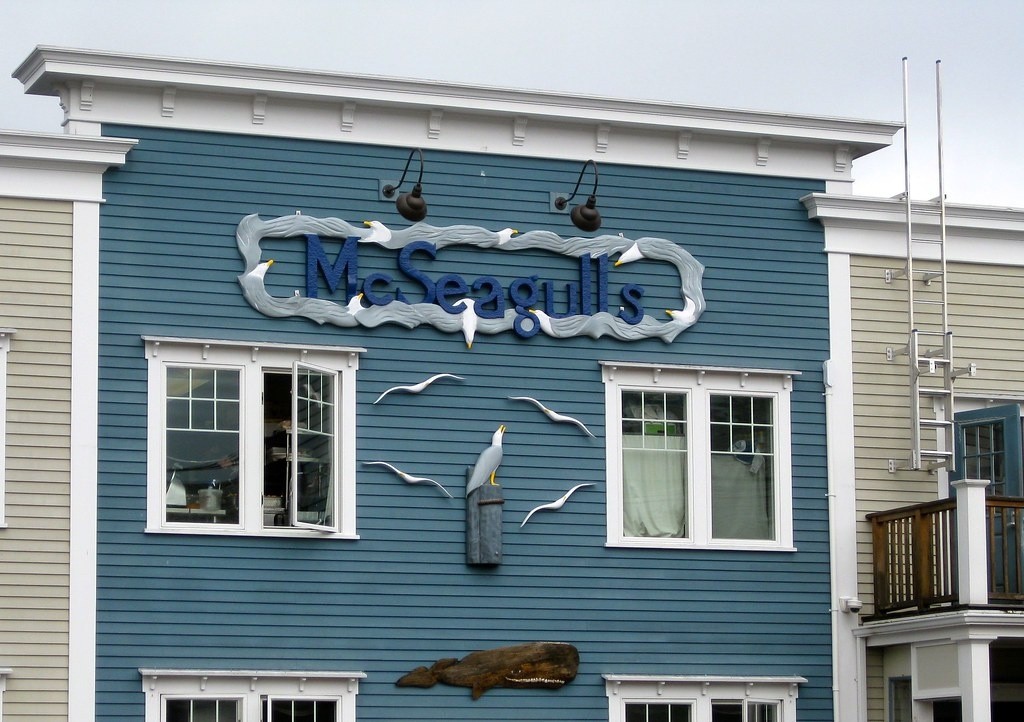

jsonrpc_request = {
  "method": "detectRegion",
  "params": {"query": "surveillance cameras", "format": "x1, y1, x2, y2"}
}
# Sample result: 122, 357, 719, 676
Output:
846, 599, 862, 613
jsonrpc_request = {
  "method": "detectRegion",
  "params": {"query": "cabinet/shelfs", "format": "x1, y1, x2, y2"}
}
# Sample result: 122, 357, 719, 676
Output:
274, 427, 329, 521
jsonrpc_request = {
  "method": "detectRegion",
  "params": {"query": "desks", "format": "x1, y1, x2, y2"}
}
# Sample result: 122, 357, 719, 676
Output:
166, 508, 226, 522
264, 507, 285, 526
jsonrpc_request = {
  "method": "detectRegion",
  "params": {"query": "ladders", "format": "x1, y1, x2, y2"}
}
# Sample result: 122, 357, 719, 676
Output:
892, 54, 975, 478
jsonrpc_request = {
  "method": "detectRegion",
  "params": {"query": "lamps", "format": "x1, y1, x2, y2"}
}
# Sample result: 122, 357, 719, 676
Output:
549, 159, 602, 232
378, 147, 428, 223
